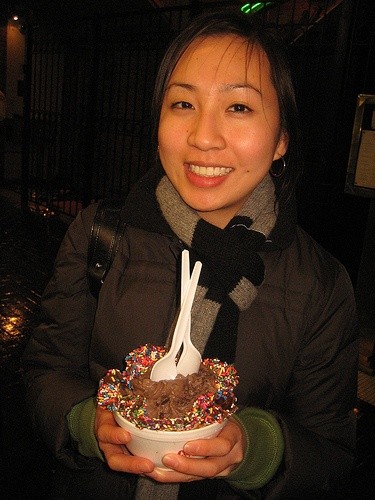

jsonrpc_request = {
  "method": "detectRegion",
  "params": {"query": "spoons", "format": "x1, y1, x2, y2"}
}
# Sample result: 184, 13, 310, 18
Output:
177, 249, 202, 378
150, 261, 202, 383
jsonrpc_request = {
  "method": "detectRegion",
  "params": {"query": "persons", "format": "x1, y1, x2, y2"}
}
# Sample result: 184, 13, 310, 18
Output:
16, 13, 359, 499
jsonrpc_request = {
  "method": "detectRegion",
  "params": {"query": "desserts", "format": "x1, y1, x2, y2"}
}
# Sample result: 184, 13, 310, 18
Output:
95, 345, 241, 432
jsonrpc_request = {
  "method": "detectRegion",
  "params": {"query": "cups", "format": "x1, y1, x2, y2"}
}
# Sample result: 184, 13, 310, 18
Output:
111, 411, 227, 473
